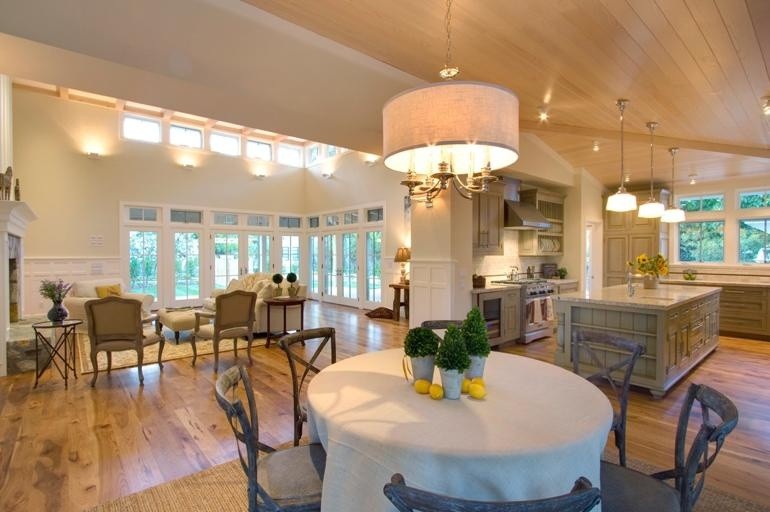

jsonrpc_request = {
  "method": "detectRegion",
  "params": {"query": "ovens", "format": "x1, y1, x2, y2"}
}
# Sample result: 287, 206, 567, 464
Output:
516, 296, 554, 344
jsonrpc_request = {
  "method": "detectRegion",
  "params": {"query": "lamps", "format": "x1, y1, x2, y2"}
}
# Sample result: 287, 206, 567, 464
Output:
394, 248, 410, 285
762, 96, 770, 114
590, 100, 685, 225
382, 0, 522, 209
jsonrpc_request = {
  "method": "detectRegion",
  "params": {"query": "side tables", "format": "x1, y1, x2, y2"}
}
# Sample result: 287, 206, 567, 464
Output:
32, 318, 83, 390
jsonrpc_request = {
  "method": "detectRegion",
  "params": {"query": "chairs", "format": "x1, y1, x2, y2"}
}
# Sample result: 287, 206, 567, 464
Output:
84, 290, 257, 388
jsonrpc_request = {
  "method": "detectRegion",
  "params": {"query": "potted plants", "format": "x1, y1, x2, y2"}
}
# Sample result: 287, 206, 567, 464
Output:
273, 274, 283, 296
40, 279, 75, 323
462, 308, 491, 379
287, 273, 297, 296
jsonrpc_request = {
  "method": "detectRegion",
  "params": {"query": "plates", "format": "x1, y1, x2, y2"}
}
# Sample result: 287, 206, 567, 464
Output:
273, 295, 290, 300
540, 224, 561, 252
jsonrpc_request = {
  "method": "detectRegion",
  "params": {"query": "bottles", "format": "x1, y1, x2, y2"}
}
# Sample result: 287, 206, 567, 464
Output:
531, 272, 534, 278
15, 178, 20, 201
527, 266, 531, 278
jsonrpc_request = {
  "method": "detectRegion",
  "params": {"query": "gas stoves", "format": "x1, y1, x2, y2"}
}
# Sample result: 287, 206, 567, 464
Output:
490, 279, 554, 297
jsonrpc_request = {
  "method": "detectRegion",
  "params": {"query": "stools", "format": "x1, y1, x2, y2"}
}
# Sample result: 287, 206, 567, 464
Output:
389, 284, 408, 321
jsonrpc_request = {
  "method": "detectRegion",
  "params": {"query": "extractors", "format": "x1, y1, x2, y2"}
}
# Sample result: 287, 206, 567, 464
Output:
505, 199, 552, 230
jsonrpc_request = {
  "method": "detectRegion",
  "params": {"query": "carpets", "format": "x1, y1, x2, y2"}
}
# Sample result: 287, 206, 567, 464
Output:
77, 332, 275, 374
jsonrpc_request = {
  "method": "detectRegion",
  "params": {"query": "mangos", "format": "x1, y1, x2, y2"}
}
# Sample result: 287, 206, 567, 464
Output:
462, 379, 470, 393
469, 384, 486, 398
415, 379, 430, 394
429, 384, 443, 399
472, 378, 484, 386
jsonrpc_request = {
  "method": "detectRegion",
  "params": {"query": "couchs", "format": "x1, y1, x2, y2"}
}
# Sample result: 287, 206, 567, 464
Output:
64, 293, 155, 336
201, 273, 307, 339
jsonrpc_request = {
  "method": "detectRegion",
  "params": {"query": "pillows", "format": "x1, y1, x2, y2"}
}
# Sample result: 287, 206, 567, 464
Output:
224, 279, 276, 302
95, 282, 121, 298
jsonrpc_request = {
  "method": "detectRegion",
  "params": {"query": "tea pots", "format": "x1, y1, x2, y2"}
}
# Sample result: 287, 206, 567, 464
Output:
505, 266, 519, 281
